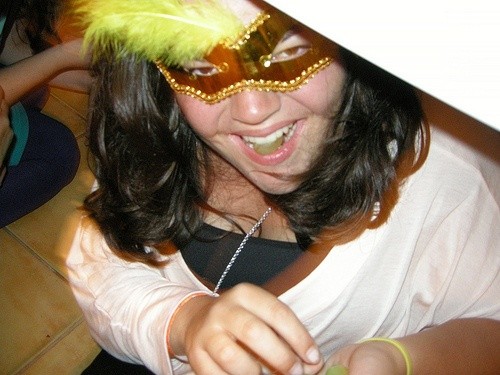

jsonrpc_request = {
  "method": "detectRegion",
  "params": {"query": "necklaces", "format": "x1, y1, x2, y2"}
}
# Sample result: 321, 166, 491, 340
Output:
209, 194, 279, 299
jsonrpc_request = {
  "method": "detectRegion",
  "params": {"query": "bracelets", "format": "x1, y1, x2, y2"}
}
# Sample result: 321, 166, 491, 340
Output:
161, 290, 210, 358
65, 4, 500, 374
352, 337, 412, 375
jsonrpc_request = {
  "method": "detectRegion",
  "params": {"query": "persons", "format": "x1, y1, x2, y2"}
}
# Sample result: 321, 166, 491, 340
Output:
0, 0, 103, 229
